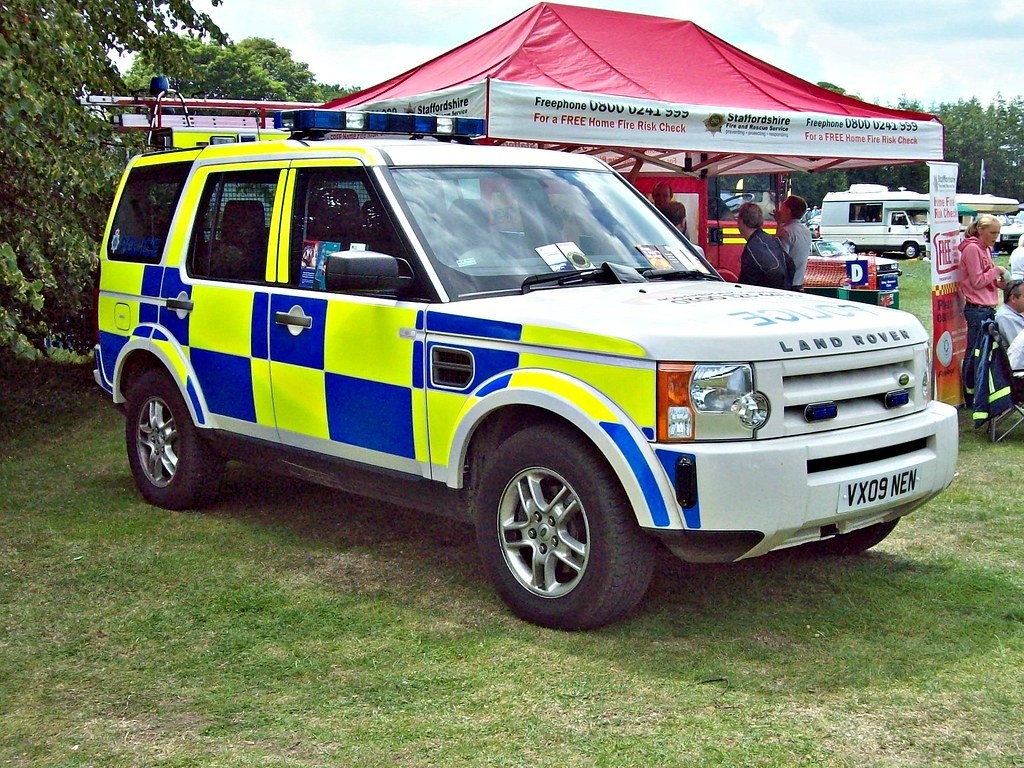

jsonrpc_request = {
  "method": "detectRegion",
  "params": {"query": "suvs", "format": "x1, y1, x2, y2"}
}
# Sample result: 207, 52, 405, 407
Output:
91, 111, 959, 630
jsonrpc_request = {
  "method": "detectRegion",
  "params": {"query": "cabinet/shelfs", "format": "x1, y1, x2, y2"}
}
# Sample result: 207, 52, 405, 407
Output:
805, 287, 899, 310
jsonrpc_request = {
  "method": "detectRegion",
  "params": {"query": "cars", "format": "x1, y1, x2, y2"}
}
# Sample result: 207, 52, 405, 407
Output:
808, 215, 820, 238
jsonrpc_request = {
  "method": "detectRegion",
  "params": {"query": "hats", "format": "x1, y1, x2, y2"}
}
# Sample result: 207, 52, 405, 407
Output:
814, 206, 817, 208
807, 208, 810, 210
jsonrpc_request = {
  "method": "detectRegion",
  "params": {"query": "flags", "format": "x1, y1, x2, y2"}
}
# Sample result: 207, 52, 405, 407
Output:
983, 165, 987, 184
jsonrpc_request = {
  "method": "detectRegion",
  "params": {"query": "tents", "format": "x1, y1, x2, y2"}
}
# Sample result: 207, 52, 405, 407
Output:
312, 3, 944, 237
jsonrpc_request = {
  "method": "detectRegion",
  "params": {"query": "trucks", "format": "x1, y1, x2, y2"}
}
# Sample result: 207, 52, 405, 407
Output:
820, 185, 926, 259
914, 192, 1024, 253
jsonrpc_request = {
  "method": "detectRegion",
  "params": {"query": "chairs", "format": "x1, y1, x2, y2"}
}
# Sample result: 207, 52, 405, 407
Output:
981, 320, 1024, 443
202, 189, 505, 282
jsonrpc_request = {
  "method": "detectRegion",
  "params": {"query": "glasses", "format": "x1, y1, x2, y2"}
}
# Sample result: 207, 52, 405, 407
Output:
734, 215, 741, 220
1007, 280, 1023, 297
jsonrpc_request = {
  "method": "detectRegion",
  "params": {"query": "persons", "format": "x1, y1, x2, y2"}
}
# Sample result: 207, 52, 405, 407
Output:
958, 214, 1008, 410
651, 181, 690, 239
995, 279, 1024, 345
1008, 234, 1024, 280
923, 224, 931, 262
660, 201, 705, 264
738, 202, 796, 291
894, 217, 903, 225
708, 190, 735, 246
805, 206, 820, 227
1006, 329, 1024, 405
769, 195, 812, 292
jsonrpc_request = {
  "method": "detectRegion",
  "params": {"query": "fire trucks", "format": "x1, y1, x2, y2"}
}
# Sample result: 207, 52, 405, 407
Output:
113, 74, 787, 283
